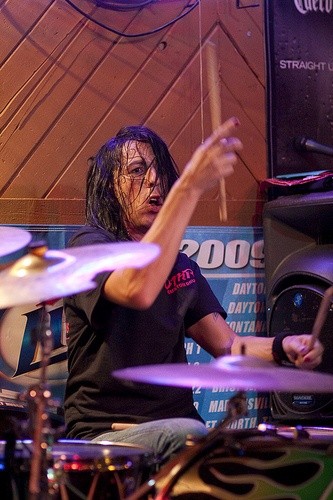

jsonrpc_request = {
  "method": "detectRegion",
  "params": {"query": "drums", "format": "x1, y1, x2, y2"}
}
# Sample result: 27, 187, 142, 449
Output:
153, 427, 333, 500
0, 441, 161, 500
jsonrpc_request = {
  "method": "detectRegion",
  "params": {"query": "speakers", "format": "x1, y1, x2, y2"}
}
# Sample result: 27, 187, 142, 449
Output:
264, 2, 333, 421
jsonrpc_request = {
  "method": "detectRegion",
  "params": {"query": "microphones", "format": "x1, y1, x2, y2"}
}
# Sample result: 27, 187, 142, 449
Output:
290, 135, 333, 157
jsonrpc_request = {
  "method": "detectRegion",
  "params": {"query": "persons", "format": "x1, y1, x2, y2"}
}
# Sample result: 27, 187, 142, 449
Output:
60, 115, 325, 483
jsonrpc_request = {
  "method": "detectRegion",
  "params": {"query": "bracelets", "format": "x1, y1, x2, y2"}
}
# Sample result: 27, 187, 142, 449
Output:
270, 332, 299, 364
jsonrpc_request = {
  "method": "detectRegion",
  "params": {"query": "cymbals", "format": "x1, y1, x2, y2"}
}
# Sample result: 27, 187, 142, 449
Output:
1, 243, 160, 309
1, 226, 32, 255
112, 355, 332, 392
